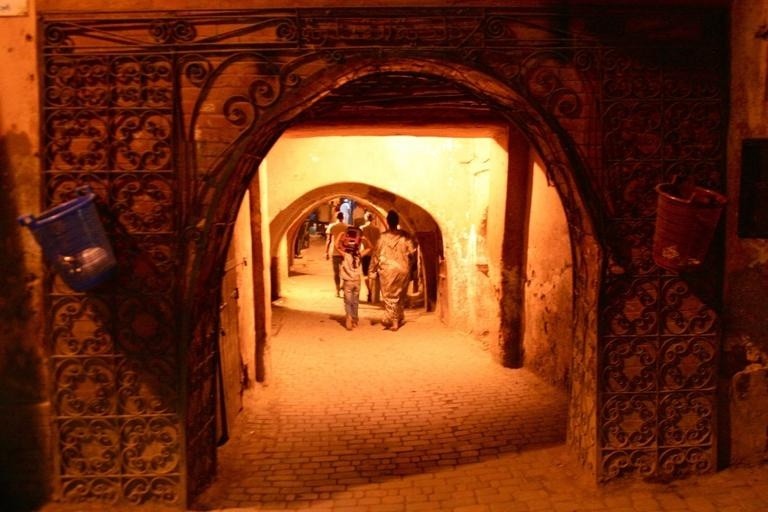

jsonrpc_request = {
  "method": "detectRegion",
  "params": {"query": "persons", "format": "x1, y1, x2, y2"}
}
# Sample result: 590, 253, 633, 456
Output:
367, 210, 416, 330
340, 199, 349, 224
325, 212, 348, 298
328, 200, 340, 212
352, 202, 365, 227
358, 212, 381, 301
334, 230, 370, 331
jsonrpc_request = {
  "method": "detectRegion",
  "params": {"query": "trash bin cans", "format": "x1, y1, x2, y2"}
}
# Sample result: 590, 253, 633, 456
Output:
18, 185, 119, 293
653, 175, 728, 274
